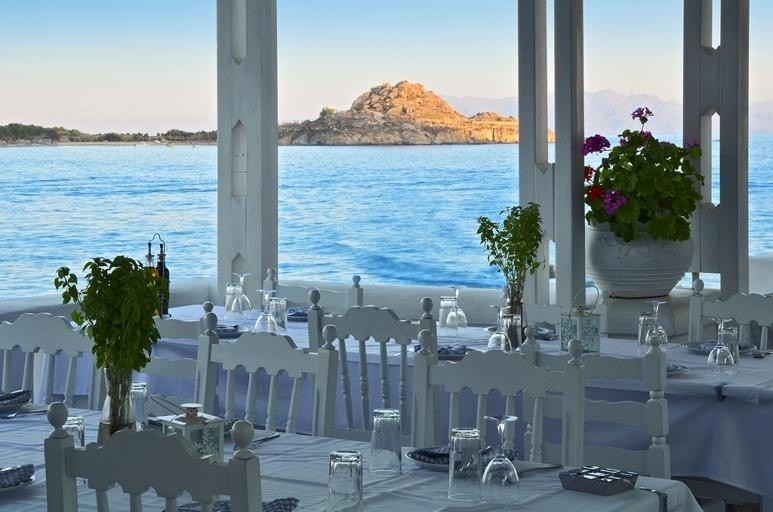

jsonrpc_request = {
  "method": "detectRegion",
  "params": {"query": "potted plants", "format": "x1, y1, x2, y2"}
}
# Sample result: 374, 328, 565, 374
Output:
473, 200, 546, 350
50, 253, 168, 440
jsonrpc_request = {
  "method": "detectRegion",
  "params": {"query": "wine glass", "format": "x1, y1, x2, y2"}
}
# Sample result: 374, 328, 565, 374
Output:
484, 304, 513, 354
645, 299, 669, 357
478, 410, 520, 507
703, 315, 736, 378
445, 286, 469, 335
250, 288, 282, 337
231, 271, 251, 316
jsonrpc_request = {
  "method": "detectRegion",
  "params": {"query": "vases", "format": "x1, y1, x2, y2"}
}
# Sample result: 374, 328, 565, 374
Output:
584, 221, 694, 297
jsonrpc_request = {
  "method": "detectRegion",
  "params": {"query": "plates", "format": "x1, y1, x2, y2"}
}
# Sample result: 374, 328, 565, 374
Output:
665, 362, 688, 379
0, 474, 35, 492
481, 325, 552, 339
681, 340, 757, 355
402, 442, 450, 470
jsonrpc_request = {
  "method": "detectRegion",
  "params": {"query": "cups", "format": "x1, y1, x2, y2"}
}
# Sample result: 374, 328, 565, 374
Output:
62, 411, 87, 488
327, 445, 363, 512
716, 323, 739, 370
130, 384, 149, 433
495, 311, 523, 351
448, 428, 483, 504
436, 294, 458, 339
224, 281, 241, 320
268, 295, 287, 332
365, 406, 403, 478
637, 310, 659, 356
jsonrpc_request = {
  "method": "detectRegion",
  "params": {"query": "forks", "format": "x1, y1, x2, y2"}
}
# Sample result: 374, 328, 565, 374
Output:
749, 349, 770, 361
1, 408, 52, 420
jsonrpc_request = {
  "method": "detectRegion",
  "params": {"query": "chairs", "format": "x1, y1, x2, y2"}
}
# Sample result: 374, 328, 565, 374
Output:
0, 292, 773, 512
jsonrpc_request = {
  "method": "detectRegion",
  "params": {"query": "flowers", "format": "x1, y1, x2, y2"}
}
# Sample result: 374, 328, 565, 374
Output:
581, 101, 706, 246
